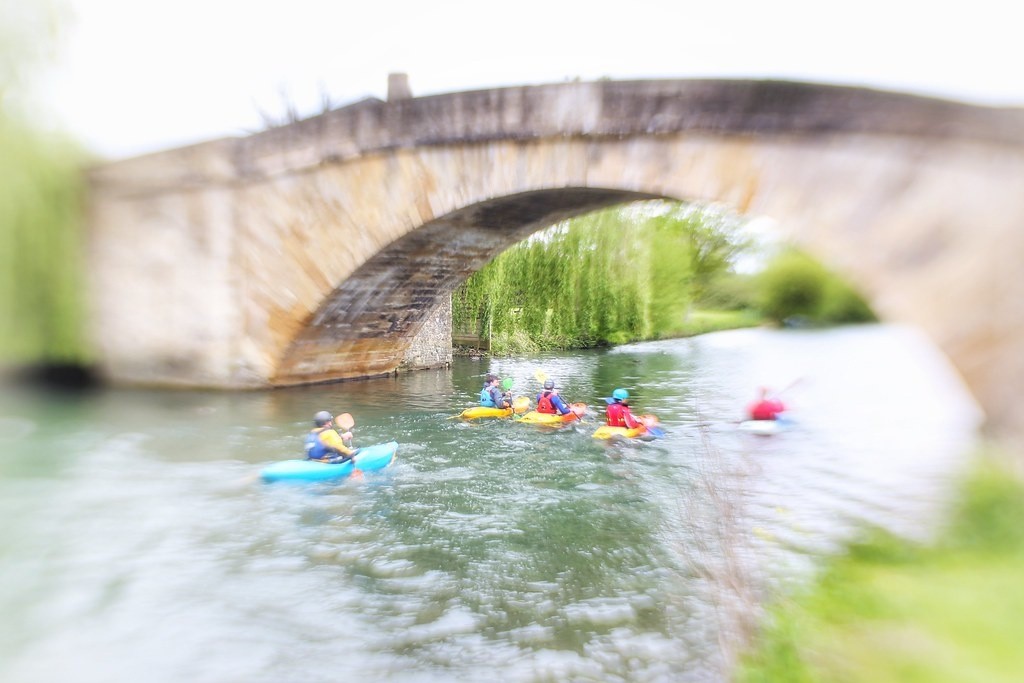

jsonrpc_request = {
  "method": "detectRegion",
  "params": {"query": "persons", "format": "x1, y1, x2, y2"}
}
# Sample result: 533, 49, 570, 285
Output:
606, 388, 646, 428
536, 378, 574, 414
750, 385, 785, 420
303, 411, 356, 464
481, 376, 512, 408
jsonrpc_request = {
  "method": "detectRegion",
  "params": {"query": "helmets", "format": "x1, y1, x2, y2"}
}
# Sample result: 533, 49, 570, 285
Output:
544, 380, 555, 389
613, 389, 629, 400
315, 411, 334, 424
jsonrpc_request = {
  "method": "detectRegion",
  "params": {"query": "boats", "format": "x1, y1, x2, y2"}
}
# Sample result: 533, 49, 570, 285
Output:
256, 441, 399, 487
736, 408, 803, 437
591, 412, 660, 441
511, 402, 586, 429
446, 396, 530, 426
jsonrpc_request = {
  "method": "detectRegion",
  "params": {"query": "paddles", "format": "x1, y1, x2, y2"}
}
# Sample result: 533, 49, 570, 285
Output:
503, 377, 520, 419
605, 397, 665, 436
533, 369, 587, 424
335, 412, 365, 482
